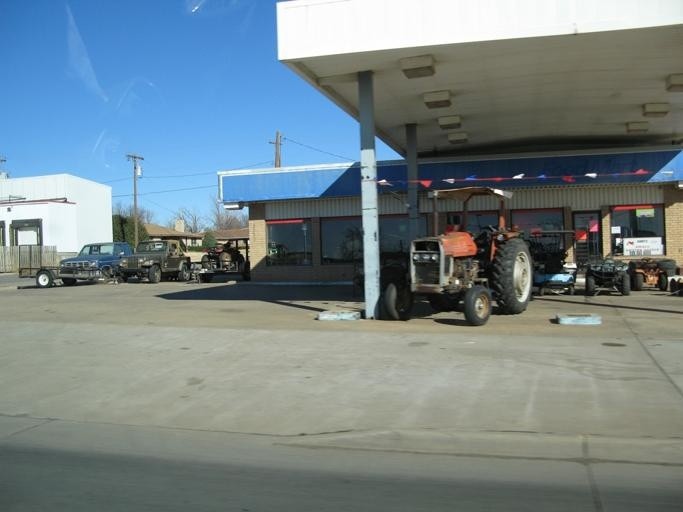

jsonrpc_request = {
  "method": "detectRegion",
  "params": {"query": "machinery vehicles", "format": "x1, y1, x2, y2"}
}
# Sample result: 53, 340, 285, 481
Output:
384, 185, 533, 325
197, 237, 250, 284
527, 229, 578, 295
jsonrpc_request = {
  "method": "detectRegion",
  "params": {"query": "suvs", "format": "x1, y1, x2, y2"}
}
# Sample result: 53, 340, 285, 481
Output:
58, 242, 134, 285
626, 257, 668, 292
581, 249, 631, 297
119, 239, 191, 283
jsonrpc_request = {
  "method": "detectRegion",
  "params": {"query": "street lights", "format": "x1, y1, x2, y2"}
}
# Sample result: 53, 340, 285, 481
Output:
300, 221, 308, 265
